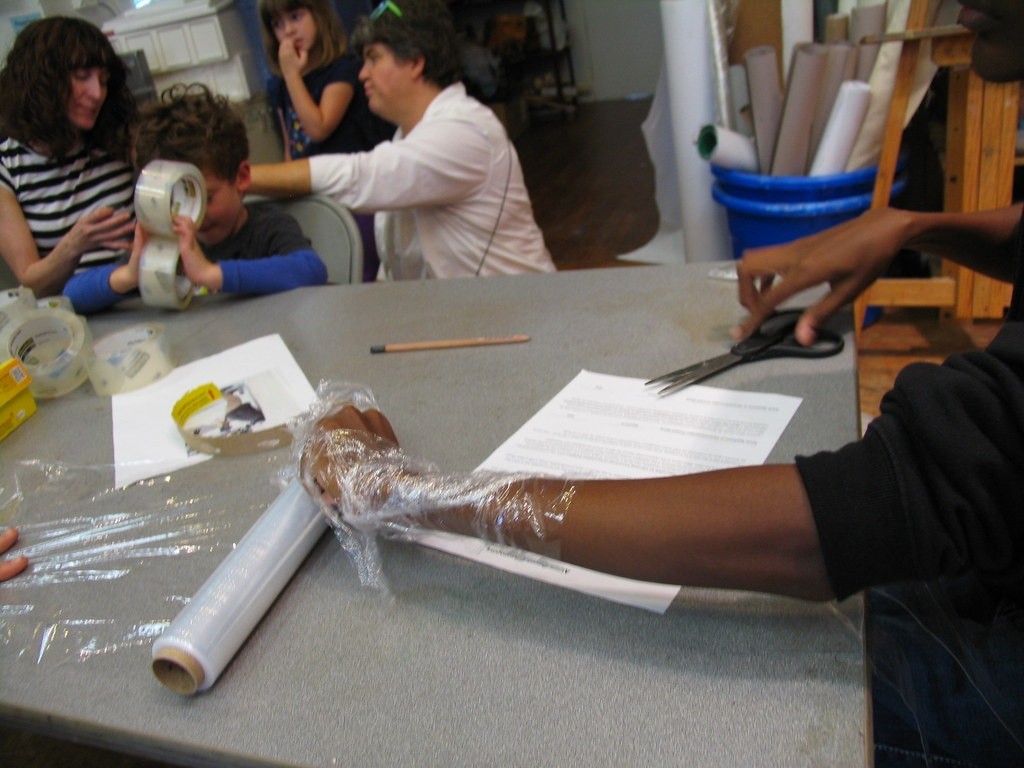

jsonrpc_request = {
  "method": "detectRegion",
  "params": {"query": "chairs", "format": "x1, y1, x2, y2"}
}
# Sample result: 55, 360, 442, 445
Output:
256, 193, 368, 284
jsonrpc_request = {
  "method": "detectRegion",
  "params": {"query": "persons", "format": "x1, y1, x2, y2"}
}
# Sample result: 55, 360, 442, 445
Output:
259, 0, 386, 285
62, 86, 326, 316
0, 17, 154, 305
297, 197, 1024, 768
0, 526, 29, 583
218, 10, 557, 282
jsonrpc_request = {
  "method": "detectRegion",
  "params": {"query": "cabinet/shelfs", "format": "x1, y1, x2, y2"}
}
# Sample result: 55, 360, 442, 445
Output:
448, 0, 578, 107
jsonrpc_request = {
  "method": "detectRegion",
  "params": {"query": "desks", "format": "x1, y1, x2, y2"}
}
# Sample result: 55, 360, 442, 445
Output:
0, 260, 874, 768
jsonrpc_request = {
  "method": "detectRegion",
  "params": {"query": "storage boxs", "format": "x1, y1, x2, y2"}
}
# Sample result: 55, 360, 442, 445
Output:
0, 358, 38, 444
486, 93, 530, 139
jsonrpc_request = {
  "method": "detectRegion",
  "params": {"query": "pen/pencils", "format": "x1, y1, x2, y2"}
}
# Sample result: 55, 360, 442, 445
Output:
370, 333, 531, 354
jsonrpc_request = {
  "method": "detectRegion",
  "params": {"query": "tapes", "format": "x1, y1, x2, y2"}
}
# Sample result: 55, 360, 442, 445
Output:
81, 322, 176, 398
138, 238, 196, 310
134, 160, 207, 238
0, 286, 92, 399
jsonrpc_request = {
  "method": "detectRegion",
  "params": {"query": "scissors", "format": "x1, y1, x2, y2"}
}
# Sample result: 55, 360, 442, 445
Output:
644, 308, 845, 395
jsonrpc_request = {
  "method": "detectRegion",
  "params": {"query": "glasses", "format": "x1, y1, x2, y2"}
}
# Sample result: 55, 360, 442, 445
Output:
369, 0, 404, 22
